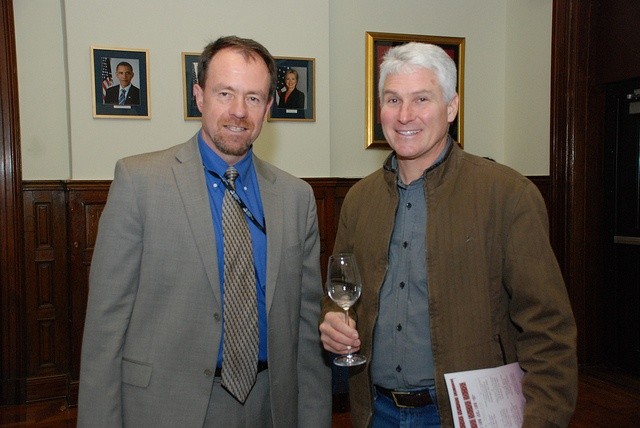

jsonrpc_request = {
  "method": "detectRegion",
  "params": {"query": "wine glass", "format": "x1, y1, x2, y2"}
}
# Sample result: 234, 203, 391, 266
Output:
326, 253, 368, 368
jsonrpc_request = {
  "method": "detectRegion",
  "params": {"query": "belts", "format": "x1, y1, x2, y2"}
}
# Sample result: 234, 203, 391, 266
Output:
375, 385, 434, 410
215, 361, 268, 377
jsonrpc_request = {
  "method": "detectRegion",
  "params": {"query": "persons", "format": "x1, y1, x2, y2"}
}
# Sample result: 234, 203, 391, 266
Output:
320, 41, 579, 427
76, 35, 333, 428
275, 69, 306, 118
104, 62, 140, 105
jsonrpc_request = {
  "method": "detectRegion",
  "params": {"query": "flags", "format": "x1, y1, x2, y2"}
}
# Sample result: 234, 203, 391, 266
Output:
101, 59, 113, 96
273, 66, 290, 105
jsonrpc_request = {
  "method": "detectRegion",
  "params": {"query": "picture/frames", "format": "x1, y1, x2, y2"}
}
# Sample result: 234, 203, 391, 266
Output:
266, 56, 316, 122
91, 46, 152, 120
367, 33, 464, 153
181, 52, 203, 120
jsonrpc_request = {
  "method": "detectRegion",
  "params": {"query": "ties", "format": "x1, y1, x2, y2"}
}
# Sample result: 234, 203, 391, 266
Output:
220, 166, 260, 405
119, 89, 126, 105
